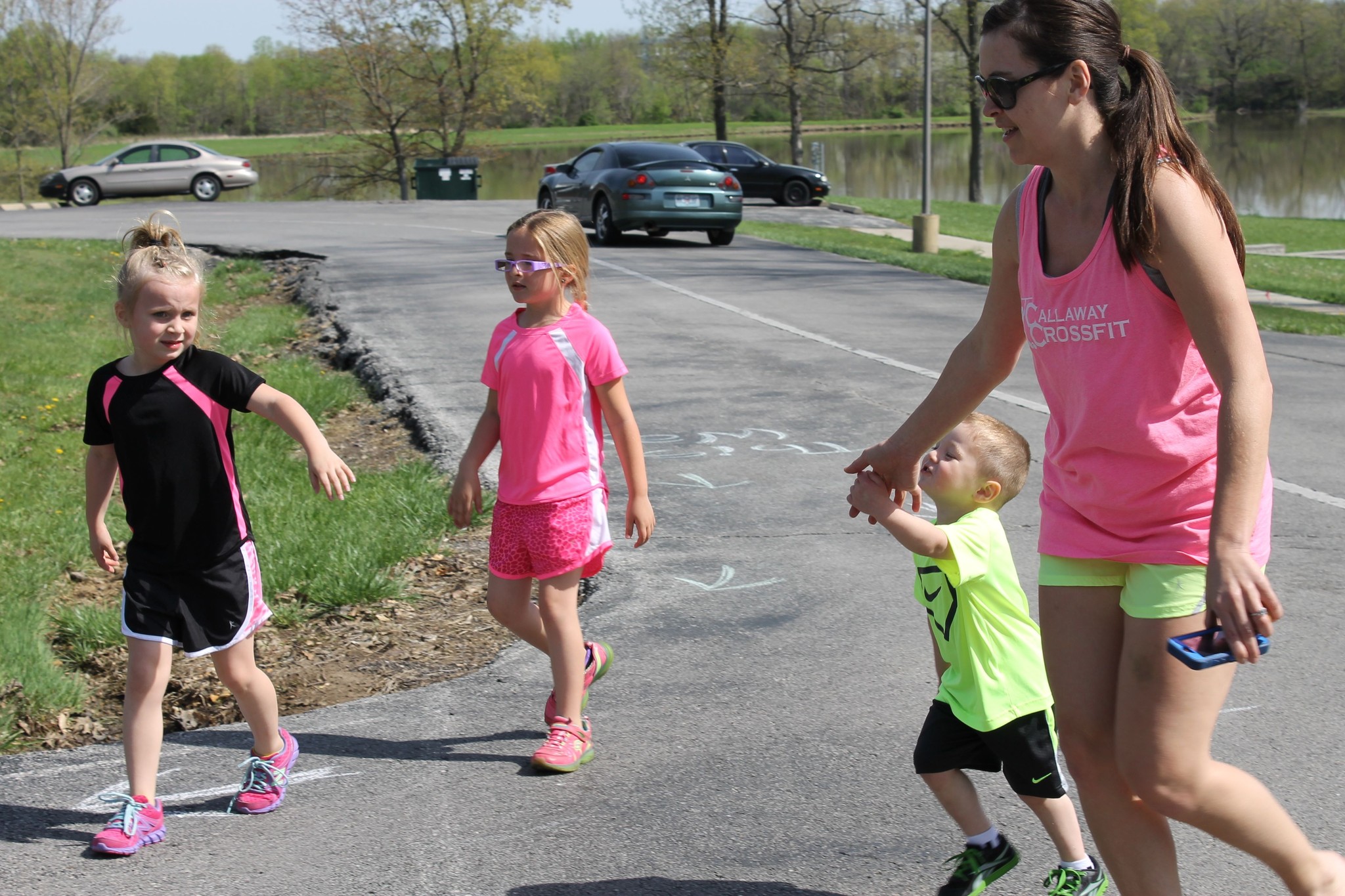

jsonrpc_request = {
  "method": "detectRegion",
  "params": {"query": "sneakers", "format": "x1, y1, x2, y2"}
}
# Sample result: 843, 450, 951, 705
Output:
938, 832, 1020, 896
1043, 855, 1108, 896
91, 791, 165, 855
530, 716, 595, 772
225, 727, 298, 816
544, 641, 613, 726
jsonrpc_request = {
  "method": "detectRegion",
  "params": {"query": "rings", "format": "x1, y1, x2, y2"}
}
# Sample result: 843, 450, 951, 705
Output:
1248, 607, 1267, 616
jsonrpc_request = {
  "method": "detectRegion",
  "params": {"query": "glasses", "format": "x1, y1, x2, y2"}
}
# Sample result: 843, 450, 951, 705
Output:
495, 259, 566, 272
974, 62, 1075, 110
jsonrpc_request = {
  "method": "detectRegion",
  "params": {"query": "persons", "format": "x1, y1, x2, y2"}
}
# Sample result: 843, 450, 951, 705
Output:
83, 216, 359, 855
842, 1, 1345, 895
845, 409, 1107, 895
449, 209, 654, 772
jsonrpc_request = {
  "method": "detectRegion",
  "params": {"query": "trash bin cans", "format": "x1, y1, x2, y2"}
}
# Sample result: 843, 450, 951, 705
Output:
414, 153, 481, 200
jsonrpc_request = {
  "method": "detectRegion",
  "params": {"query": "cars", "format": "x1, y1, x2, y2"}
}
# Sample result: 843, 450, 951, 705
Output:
37, 137, 259, 206
536, 137, 744, 247
678, 139, 831, 207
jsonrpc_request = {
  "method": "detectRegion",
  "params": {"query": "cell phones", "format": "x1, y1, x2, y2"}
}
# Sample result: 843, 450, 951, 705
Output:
1166, 623, 1271, 671
